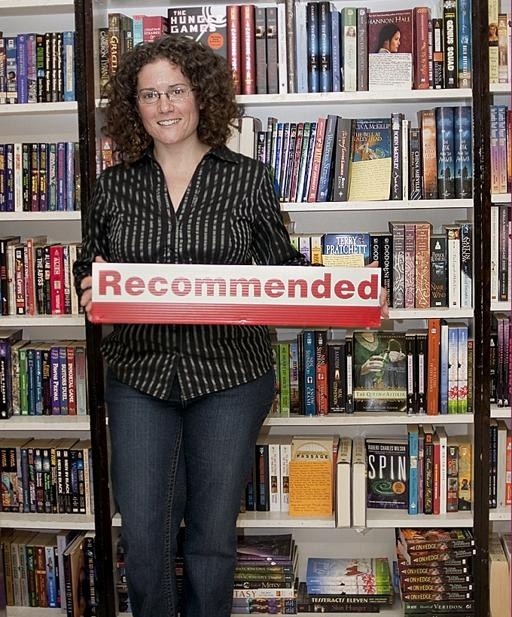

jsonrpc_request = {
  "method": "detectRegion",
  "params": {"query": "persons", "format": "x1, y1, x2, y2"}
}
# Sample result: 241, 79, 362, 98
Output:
72, 33, 387, 617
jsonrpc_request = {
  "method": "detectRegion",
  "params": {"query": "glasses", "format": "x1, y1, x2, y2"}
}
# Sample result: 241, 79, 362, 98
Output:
135, 85, 191, 104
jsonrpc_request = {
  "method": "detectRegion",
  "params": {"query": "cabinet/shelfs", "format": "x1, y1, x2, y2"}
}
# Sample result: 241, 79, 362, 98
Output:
0, 1, 512, 617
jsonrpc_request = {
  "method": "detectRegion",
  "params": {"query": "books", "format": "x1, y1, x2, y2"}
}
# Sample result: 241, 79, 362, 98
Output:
281, 206, 512, 309
239, 418, 512, 528
114, 527, 511, 616
1, 528, 99, 617
1, 234, 85, 315
1, 437, 95, 516
0, 31, 75, 104
270, 314, 512, 417
0, 142, 80, 212
1, 329, 90, 419
96, 1, 512, 102
226, 105, 512, 202
